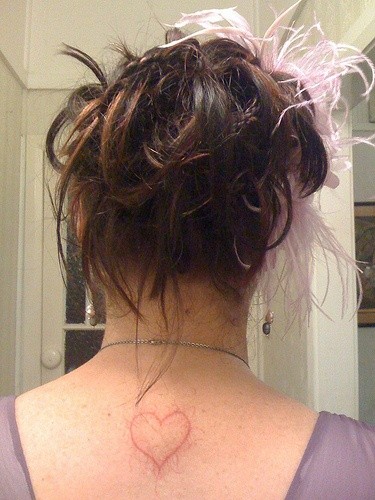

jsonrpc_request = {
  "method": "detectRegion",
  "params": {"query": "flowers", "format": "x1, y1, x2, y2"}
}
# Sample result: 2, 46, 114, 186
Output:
164, 1, 375, 326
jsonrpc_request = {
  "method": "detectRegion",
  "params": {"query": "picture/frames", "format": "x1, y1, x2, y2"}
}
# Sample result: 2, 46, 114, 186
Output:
354, 202, 375, 327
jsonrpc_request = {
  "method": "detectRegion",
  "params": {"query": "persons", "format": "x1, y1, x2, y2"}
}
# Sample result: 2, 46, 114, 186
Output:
0, 1, 375, 500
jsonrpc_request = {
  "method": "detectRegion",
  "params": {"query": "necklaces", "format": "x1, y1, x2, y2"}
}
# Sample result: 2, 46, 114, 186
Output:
96, 337, 249, 367
